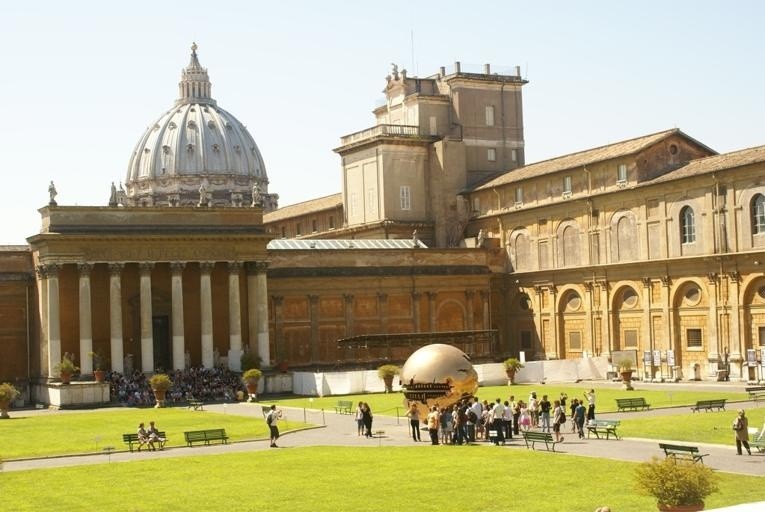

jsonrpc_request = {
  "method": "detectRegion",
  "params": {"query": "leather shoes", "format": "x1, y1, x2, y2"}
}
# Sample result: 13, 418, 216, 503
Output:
747, 449, 751, 455
735, 452, 742, 455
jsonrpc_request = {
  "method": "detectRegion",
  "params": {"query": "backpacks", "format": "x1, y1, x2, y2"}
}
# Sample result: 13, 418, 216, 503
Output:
560, 411, 567, 423
267, 413, 273, 424
469, 411, 477, 424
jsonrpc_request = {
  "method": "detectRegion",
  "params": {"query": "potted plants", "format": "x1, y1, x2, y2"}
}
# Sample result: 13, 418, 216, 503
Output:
276, 348, 291, 373
620, 357, 634, 381
376, 364, 401, 386
58, 351, 80, 381
0, 381, 20, 410
146, 371, 171, 399
242, 366, 264, 394
87, 349, 107, 379
505, 357, 520, 378
635, 454, 718, 512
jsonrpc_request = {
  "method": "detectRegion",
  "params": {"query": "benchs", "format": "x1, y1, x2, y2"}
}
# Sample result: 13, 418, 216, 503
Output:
693, 396, 728, 413
261, 405, 273, 418
746, 385, 765, 402
614, 397, 652, 412
657, 441, 703, 466
518, 429, 560, 453
585, 416, 619, 439
122, 431, 168, 453
186, 398, 204, 411
335, 398, 353, 416
747, 432, 765, 453
184, 427, 229, 448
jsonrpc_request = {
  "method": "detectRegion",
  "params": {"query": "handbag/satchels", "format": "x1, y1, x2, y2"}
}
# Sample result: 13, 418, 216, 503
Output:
732, 422, 743, 430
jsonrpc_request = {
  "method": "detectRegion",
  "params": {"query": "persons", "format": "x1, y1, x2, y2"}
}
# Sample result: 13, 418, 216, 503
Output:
137, 423, 151, 451
109, 181, 117, 203
266, 405, 282, 447
145, 421, 169, 451
732, 409, 752, 456
48, 180, 58, 201
198, 185, 207, 203
105, 363, 247, 407
251, 181, 263, 207
355, 389, 596, 446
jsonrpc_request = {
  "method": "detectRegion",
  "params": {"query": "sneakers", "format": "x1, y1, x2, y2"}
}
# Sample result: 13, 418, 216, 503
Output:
271, 444, 273, 447
272, 443, 277, 447
